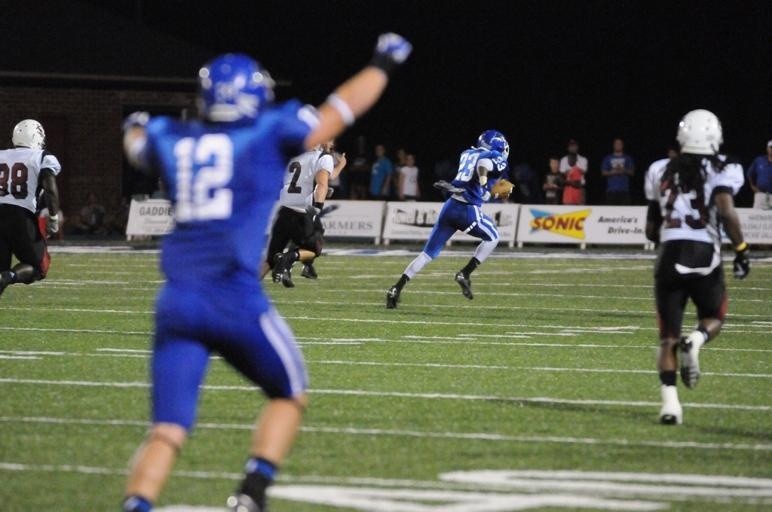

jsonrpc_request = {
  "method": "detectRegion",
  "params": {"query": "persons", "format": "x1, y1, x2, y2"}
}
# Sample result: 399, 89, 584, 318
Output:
367, 144, 421, 200
667, 145, 679, 158
384, 127, 514, 309
513, 139, 632, 205
38, 202, 67, 238
271, 136, 347, 282
0, 116, 60, 292
119, 31, 415, 511
260, 134, 333, 288
640, 109, 753, 429
747, 140, 771, 209
71, 190, 110, 236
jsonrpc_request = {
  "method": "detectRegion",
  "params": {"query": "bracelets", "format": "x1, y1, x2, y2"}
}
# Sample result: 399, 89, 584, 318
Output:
730, 238, 749, 256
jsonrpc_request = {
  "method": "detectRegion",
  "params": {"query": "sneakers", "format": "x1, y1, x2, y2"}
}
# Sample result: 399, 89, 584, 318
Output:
455, 271, 473, 299
680, 334, 699, 387
659, 403, 683, 424
387, 287, 398, 308
272, 253, 317, 287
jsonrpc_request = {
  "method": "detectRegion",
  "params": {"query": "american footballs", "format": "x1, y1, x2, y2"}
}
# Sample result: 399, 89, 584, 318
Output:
491, 180, 513, 197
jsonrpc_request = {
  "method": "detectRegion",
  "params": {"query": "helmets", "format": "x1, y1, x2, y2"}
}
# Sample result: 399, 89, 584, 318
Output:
477, 130, 509, 160
12, 118, 45, 149
199, 53, 275, 123
676, 109, 723, 156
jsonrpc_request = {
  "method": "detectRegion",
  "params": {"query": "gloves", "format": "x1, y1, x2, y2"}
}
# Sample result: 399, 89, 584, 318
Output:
733, 246, 749, 278
375, 33, 412, 74
47, 214, 59, 233
